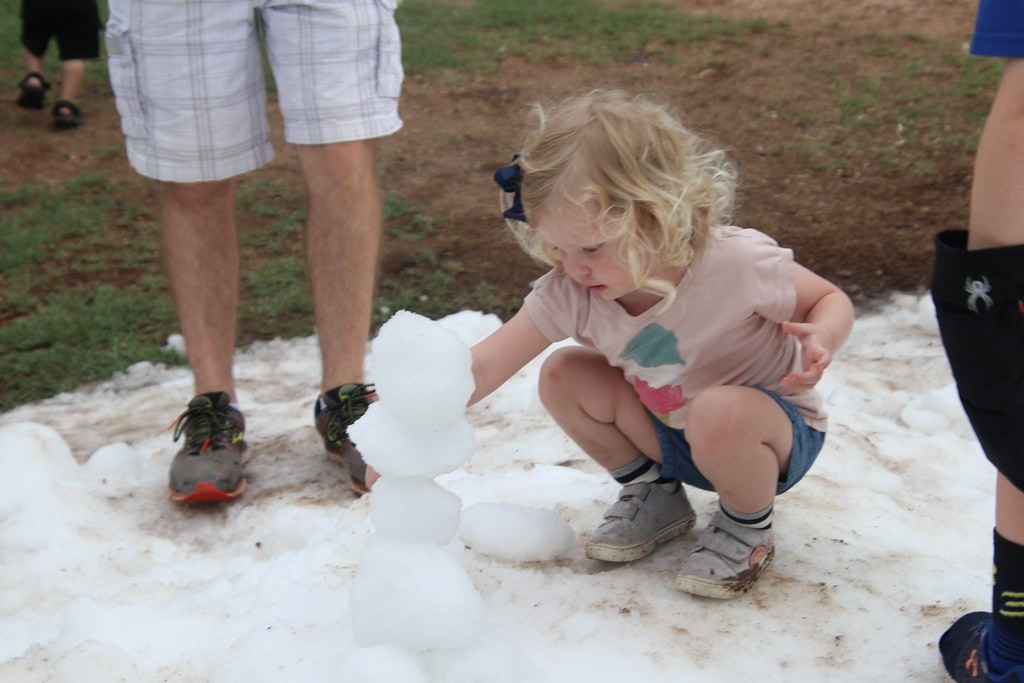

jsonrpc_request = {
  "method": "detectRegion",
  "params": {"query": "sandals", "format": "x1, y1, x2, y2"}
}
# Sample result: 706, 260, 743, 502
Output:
16, 72, 51, 109
53, 99, 82, 129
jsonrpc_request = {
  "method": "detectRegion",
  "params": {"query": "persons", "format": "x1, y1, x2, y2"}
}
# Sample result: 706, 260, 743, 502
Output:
101, 0, 402, 502
362, 90, 856, 599
17, 0, 102, 122
939, 1, 1024, 683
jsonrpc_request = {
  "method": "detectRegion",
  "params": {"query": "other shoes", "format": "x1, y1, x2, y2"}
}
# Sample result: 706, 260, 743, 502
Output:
939, 610, 1024, 683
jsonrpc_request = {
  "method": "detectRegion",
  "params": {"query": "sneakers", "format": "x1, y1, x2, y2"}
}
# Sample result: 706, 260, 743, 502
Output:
315, 382, 385, 498
164, 392, 246, 502
583, 481, 696, 563
675, 509, 776, 599
